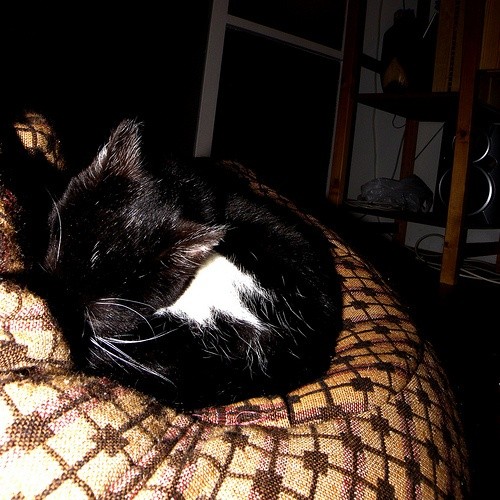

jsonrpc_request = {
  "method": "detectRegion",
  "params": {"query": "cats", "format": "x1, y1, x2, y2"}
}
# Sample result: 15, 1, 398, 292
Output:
39, 117, 342, 410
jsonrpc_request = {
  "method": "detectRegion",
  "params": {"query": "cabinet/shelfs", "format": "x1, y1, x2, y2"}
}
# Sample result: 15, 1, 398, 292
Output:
199, 0, 498, 289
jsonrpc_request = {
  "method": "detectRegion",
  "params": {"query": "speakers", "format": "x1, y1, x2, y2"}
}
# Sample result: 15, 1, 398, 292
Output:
434, 123, 500, 225
